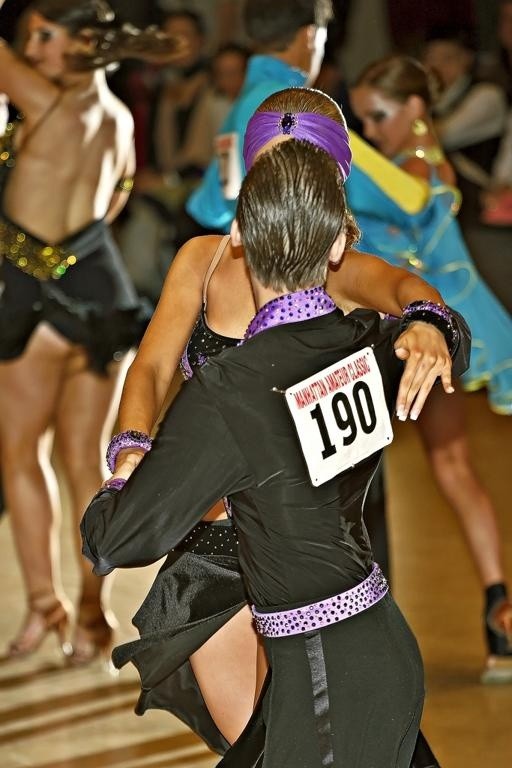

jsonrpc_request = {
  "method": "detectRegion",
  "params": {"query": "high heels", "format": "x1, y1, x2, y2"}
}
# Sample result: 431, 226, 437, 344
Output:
67, 595, 116, 668
1, 590, 69, 665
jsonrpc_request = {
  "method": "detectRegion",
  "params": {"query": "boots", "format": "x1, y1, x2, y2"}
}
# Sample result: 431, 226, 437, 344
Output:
484, 584, 510, 655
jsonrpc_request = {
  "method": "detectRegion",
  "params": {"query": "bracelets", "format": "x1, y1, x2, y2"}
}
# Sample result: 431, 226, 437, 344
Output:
400, 300, 461, 359
103, 430, 157, 472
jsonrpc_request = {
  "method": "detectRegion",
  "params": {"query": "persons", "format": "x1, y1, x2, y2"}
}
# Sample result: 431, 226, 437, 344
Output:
97, 84, 460, 768
1, 0, 511, 685
77, 141, 470, 768
